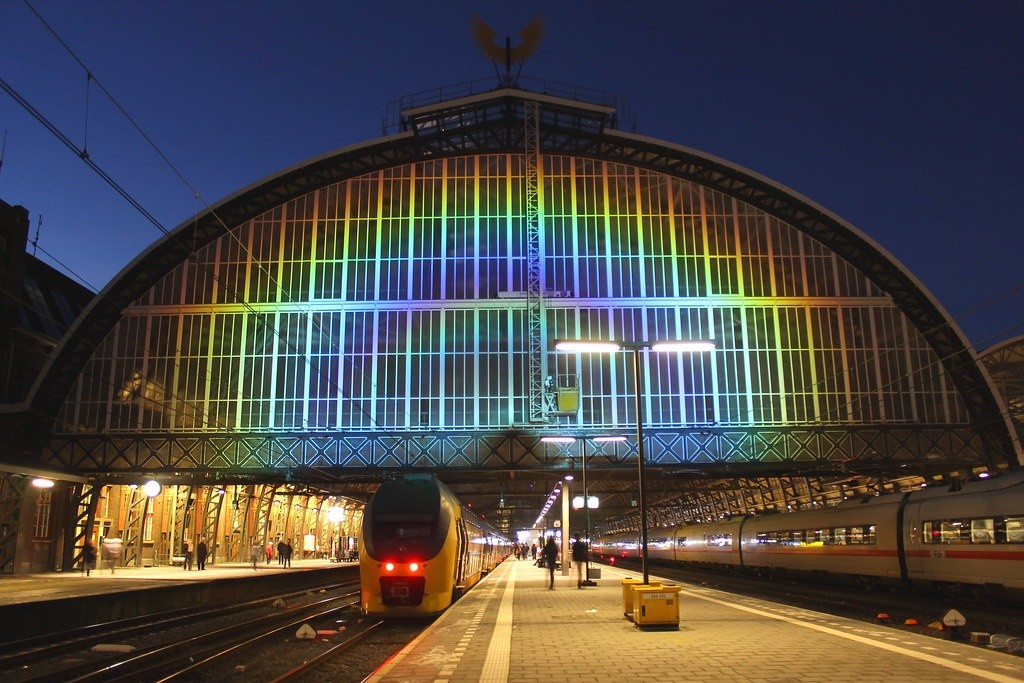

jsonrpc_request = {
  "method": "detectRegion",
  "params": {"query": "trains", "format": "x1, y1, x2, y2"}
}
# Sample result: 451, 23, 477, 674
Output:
587, 472, 1023, 591
359, 471, 516, 619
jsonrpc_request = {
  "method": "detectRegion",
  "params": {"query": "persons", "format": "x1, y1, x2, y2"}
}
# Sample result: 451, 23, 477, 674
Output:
80, 538, 123, 577
266, 541, 274, 565
196, 537, 207, 571
330, 537, 358, 562
533, 535, 559, 591
252, 539, 293, 571
572, 531, 588, 588
183, 539, 193, 572
511, 541, 537, 561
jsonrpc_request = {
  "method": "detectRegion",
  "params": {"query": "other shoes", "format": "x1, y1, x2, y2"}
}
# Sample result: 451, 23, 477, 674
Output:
202, 568, 206, 570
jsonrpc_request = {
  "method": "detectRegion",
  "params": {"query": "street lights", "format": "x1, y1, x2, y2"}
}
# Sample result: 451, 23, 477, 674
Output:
552, 336, 718, 585
541, 435, 628, 586
